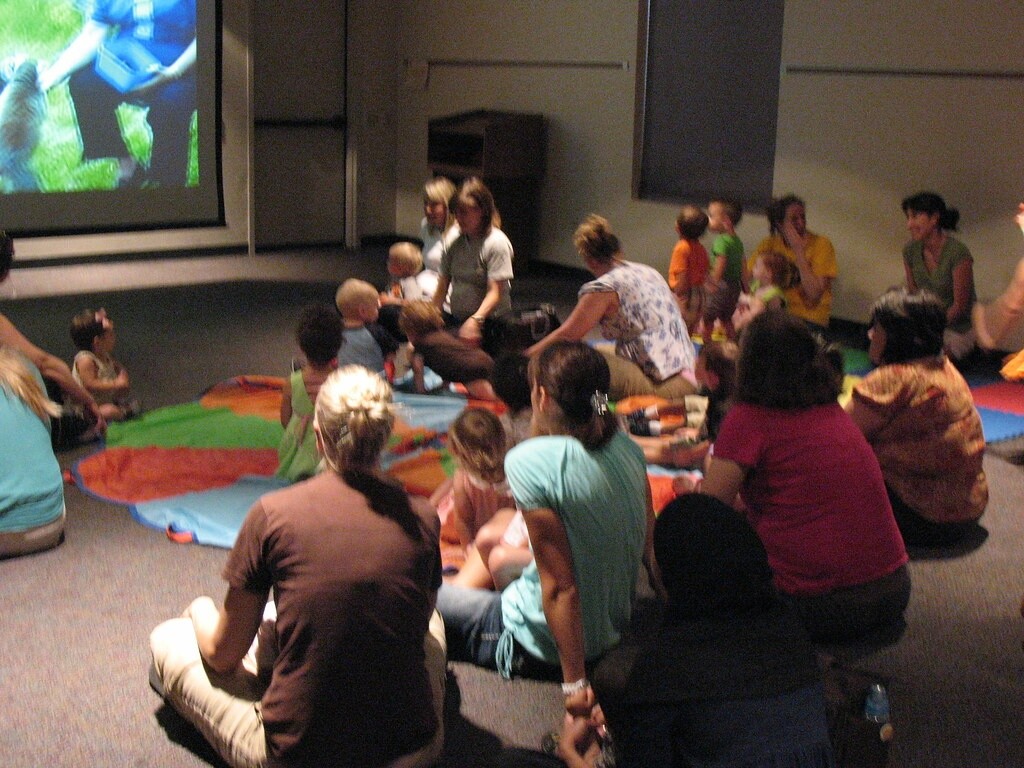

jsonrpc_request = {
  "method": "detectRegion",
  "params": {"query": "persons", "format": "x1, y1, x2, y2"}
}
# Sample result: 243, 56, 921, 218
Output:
701, 196, 754, 344
40, 0, 198, 192
146, 363, 449, 768
520, 212, 701, 400
436, 340, 674, 718
64, 306, 136, 423
269, 300, 348, 484
0, 175, 1024, 768
901, 190, 978, 361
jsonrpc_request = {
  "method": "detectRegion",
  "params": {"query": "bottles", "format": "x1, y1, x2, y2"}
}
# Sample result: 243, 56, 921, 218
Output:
863, 685, 890, 726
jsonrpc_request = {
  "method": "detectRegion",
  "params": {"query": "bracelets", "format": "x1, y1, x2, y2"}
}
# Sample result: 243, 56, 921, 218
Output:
560, 675, 590, 694
471, 314, 485, 325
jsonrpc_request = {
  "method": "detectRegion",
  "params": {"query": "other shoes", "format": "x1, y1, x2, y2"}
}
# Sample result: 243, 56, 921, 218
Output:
629, 417, 652, 437
116, 162, 146, 190
624, 408, 645, 419
